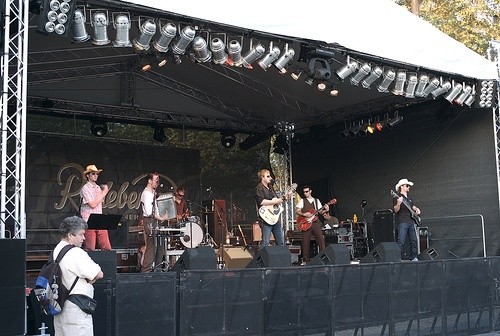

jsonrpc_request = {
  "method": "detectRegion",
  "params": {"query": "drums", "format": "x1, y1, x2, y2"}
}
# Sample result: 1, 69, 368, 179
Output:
173, 221, 203, 249
186, 216, 200, 224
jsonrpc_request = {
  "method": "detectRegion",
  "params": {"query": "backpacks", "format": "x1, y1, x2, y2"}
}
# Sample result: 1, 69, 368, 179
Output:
30, 244, 80, 316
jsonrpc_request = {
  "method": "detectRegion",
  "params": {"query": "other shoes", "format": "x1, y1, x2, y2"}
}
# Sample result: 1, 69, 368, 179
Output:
300, 261, 307, 266
411, 258, 418, 261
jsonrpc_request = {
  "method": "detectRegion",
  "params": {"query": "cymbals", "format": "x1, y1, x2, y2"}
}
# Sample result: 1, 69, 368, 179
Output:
199, 211, 215, 215
201, 203, 215, 207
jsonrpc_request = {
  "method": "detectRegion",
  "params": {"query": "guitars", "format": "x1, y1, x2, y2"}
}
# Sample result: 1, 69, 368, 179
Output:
297, 199, 337, 232
257, 182, 298, 227
390, 189, 423, 227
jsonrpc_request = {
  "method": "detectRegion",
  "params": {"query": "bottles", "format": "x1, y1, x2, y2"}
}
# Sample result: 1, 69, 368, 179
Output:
354, 214, 357, 224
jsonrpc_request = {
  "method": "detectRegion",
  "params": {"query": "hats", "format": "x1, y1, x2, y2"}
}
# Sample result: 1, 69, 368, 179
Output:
82, 165, 103, 180
395, 178, 414, 191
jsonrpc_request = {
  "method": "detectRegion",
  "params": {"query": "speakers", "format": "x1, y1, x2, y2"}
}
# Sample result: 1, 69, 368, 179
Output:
86, 250, 117, 274
203, 200, 226, 246
171, 247, 219, 270
96, 221, 129, 249
245, 246, 292, 267
417, 242, 448, 261
395, 226, 429, 259
307, 244, 351, 265
374, 209, 396, 246
359, 242, 401, 263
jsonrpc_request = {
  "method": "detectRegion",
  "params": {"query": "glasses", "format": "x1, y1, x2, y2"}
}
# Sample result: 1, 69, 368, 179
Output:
92, 172, 99, 175
267, 175, 269, 178
178, 192, 185, 197
154, 180, 160, 183
303, 191, 308, 193
406, 184, 410, 188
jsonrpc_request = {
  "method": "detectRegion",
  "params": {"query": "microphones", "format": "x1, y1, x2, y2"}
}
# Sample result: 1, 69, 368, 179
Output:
186, 200, 192, 205
270, 178, 277, 184
207, 189, 213, 193
160, 184, 173, 190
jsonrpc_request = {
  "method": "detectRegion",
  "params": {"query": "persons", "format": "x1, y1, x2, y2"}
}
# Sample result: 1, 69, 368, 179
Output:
322, 212, 339, 244
254, 169, 284, 257
80, 164, 111, 251
169, 187, 190, 245
295, 185, 329, 265
141, 171, 168, 273
53, 216, 103, 336
393, 179, 420, 261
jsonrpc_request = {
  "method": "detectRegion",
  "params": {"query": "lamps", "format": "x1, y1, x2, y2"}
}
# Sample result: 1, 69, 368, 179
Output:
349, 60, 372, 86
424, 73, 440, 97
444, 79, 463, 105
362, 62, 383, 89
339, 109, 405, 140
89, 8, 111, 45
90, 122, 109, 137
71, 5, 90, 42
391, 69, 407, 96
111, 12, 132, 48
464, 84, 477, 107
220, 133, 236, 148
38, 0, 76, 38
375, 66, 395, 93
404, 72, 418, 98
132, 15, 340, 97
415, 71, 429, 97
454, 81, 471, 105
431, 76, 452, 98
334, 55, 359, 82
